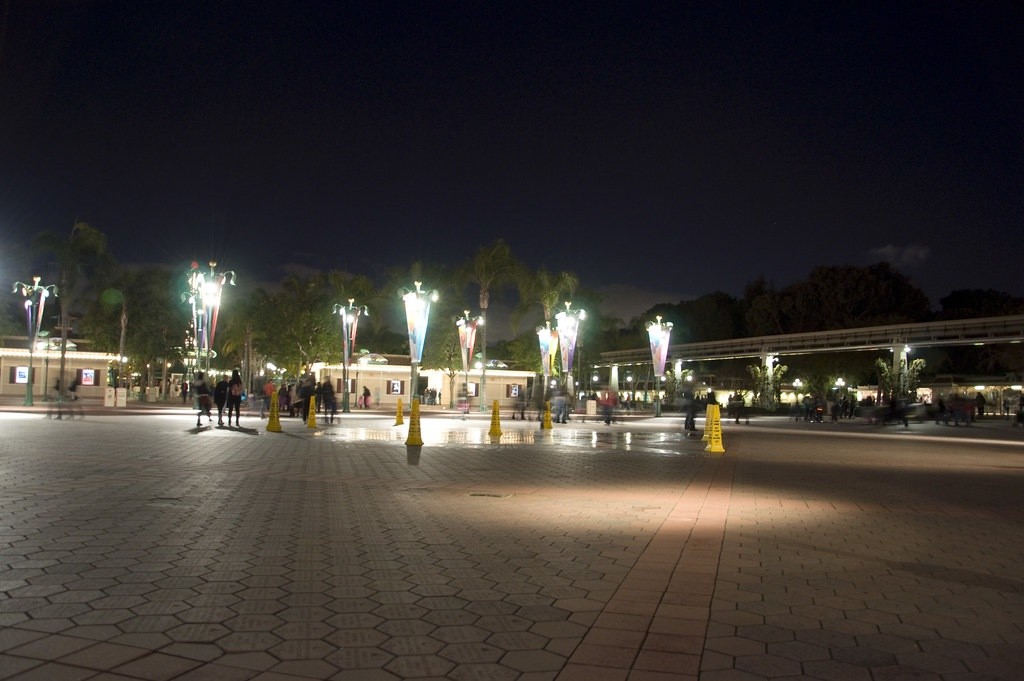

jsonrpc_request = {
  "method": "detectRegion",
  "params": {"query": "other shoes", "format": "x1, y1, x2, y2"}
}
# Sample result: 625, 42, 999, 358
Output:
190, 417, 244, 430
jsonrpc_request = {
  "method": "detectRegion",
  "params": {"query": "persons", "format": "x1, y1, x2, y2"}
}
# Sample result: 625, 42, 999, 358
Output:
228, 369, 244, 426
358, 386, 380, 409
213, 375, 229, 425
425, 387, 441, 406
264, 375, 338, 424
801, 388, 1011, 427
68, 378, 79, 401
155, 378, 216, 403
683, 381, 749, 431
52, 377, 60, 403
512, 385, 646, 425
194, 373, 213, 426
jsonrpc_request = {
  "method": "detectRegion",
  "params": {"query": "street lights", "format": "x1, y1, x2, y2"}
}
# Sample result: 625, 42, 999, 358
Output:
450, 310, 484, 414
534, 320, 559, 388
645, 315, 674, 417
397, 280, 440, 405
554, 302, 586, 393
180, 260, 237, 411
792, 379, 803, 402
331, 298, 369, 413
835, 378, 846, 392
10, 277, 59, 408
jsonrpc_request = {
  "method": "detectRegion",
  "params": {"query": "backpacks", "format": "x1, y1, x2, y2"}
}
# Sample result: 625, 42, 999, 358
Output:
231, 381, 243, 395
